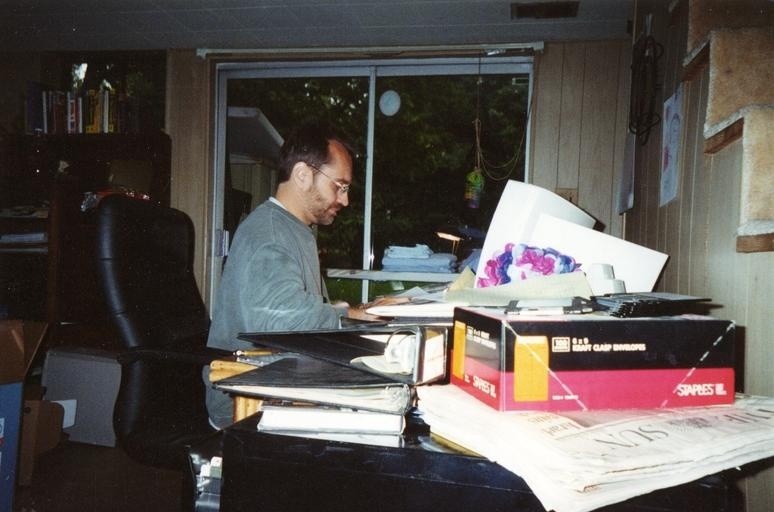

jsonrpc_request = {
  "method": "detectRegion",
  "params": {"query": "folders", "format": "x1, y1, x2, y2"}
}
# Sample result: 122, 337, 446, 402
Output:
236, 326, 454, 385
212, 356, 418, 415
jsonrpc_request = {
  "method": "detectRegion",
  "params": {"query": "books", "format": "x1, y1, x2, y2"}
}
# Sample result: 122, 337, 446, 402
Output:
255, 401, 407, 450
22, 78, 127, 135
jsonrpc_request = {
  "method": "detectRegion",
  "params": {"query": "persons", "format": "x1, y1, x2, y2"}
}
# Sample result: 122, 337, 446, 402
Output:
200, 127, 409, 434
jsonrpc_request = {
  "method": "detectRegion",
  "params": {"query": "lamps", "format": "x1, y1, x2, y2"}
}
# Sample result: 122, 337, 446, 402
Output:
436, 226, 461, 254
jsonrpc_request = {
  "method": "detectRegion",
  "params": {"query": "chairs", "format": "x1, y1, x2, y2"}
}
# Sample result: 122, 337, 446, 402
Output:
95, 194, 212, 512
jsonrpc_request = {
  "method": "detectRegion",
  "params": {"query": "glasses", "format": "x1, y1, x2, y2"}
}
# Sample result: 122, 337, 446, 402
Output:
309, 164, 351, 194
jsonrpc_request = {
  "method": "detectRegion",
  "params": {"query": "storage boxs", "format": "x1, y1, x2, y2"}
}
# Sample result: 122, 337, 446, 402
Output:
450, 304, 735, 413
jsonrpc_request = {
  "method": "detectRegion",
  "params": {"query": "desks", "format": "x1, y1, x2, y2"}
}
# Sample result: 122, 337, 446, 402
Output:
221, 411, 727, 512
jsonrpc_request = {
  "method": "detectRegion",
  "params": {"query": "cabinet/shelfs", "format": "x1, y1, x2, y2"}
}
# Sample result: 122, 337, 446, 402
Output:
1, 46, 171, 346
